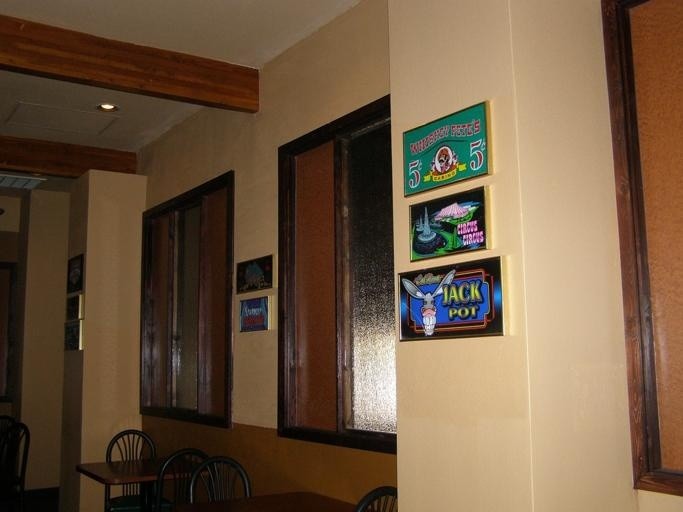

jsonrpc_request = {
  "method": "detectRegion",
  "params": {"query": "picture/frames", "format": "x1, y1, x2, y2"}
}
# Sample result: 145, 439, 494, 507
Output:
399, 101, 505, 344
235, 254, 273, 334
66, 254, 84, 294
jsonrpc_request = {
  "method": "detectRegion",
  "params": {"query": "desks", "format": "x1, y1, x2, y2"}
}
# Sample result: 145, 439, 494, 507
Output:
75, 455, 210, 511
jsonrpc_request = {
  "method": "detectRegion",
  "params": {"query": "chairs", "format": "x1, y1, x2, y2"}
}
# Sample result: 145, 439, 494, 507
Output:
105, 429, 396, 511
0, 415, 32, 511
